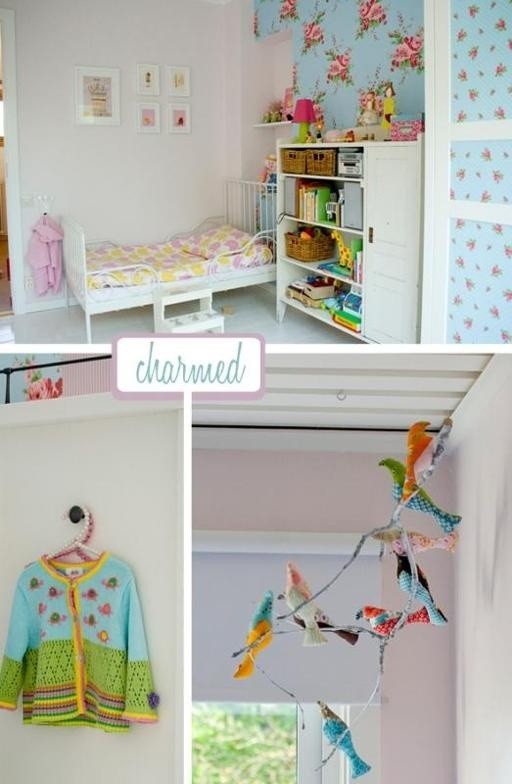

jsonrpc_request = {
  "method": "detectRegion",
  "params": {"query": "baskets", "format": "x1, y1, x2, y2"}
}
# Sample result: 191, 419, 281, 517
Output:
280, 149, 336, 176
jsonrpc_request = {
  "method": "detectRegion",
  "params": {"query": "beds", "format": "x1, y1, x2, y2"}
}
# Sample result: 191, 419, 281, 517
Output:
62, 178, 277, 344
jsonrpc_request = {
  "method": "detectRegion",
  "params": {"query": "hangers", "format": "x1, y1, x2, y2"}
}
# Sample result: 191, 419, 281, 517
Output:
45, 504, 102, 564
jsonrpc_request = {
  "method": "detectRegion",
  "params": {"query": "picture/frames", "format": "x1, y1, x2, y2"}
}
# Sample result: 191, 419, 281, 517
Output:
282, 87, 293, 122
166, 101, 190, 134
136, 63, 160, 96
137, 102, 161, 133
73, 66, 120, 127
165, 64, 190, 97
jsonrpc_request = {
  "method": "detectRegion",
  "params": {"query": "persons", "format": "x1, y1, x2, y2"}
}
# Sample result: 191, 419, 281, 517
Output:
286, 224, 364, 336
258, 153, 278, 201
381, 83, 397, 139
361, 92, 379, 138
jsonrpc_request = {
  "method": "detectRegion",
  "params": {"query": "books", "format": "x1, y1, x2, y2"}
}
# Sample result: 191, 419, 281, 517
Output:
292, 176, 344, 230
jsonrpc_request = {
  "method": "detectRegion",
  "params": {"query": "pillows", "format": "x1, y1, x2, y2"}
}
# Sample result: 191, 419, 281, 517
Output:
182, 223, 262, 261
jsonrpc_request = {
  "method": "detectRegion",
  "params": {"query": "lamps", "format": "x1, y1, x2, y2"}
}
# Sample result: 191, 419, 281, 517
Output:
291, 99, 318, 143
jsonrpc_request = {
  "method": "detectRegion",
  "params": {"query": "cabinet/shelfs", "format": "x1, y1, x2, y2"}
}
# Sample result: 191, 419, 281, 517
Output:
274, 139, 422, 343
